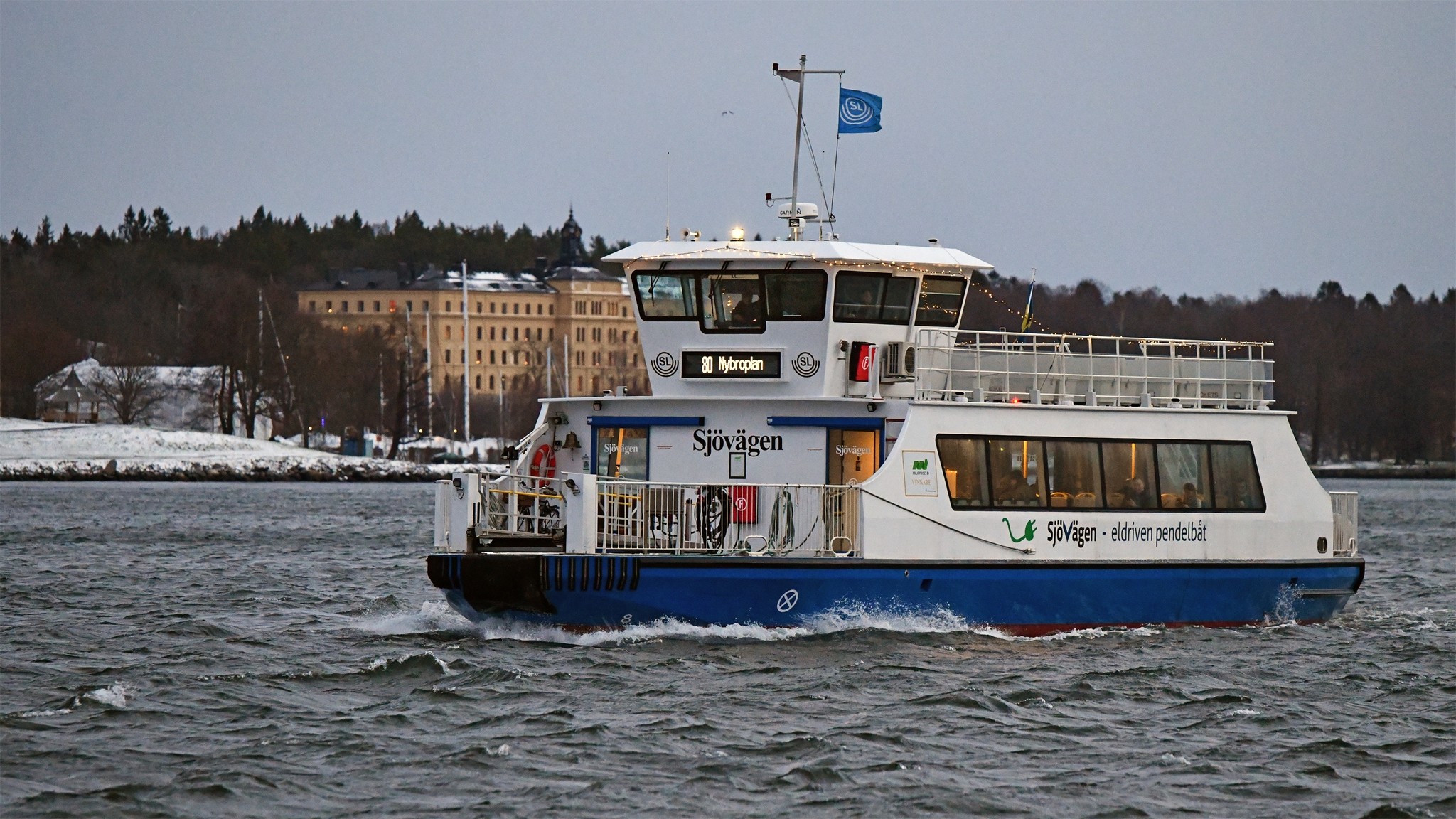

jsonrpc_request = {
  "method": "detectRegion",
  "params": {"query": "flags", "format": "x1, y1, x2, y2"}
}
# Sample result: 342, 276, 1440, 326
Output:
837, 88, 883, 134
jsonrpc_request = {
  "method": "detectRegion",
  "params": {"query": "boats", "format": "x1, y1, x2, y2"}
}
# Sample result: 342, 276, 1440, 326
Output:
422, 32, 1371, 639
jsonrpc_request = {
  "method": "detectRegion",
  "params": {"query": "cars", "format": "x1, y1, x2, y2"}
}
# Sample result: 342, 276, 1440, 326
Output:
431, 453, 469, 464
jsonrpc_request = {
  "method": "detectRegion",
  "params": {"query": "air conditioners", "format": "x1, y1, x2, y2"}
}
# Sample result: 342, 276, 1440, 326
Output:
886, 341, 920, 377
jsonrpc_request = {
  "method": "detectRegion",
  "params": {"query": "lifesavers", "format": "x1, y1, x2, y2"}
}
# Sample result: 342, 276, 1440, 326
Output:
530, 444, 557, 488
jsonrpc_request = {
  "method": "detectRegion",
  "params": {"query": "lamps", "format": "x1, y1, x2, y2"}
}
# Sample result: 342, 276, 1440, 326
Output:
562, 430, 582, 462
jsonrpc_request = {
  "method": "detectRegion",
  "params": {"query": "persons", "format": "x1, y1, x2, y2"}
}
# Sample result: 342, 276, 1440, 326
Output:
731, 286, 877, 326
997, 467, 1259, 510
